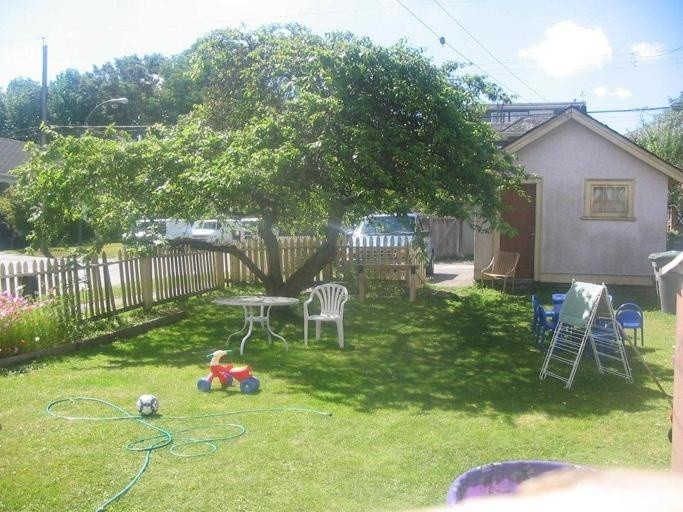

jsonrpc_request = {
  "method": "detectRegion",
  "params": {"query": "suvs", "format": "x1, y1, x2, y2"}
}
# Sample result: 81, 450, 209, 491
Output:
342, 210, 434, 279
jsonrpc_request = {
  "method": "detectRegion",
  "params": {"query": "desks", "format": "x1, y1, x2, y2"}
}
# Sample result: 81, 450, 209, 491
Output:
215, 294, 298, 355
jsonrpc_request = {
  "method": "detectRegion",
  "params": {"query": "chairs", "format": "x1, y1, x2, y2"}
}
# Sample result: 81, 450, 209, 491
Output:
479, 250, 519, 292
530, 292, 648, 357
302, 283, 349, 348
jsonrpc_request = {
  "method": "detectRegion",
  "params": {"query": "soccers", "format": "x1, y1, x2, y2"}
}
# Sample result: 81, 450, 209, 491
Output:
137, 395, 159, 416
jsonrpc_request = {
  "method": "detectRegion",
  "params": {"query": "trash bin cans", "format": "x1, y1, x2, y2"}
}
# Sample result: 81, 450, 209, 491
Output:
445, 460, 610, 509
649, 250, 683, 314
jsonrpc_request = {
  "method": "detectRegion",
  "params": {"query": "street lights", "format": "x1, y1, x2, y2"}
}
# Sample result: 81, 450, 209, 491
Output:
74, 95, 130, 246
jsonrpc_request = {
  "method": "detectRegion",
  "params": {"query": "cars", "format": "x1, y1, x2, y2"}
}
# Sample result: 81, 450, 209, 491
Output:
119, 212, 282, 251
